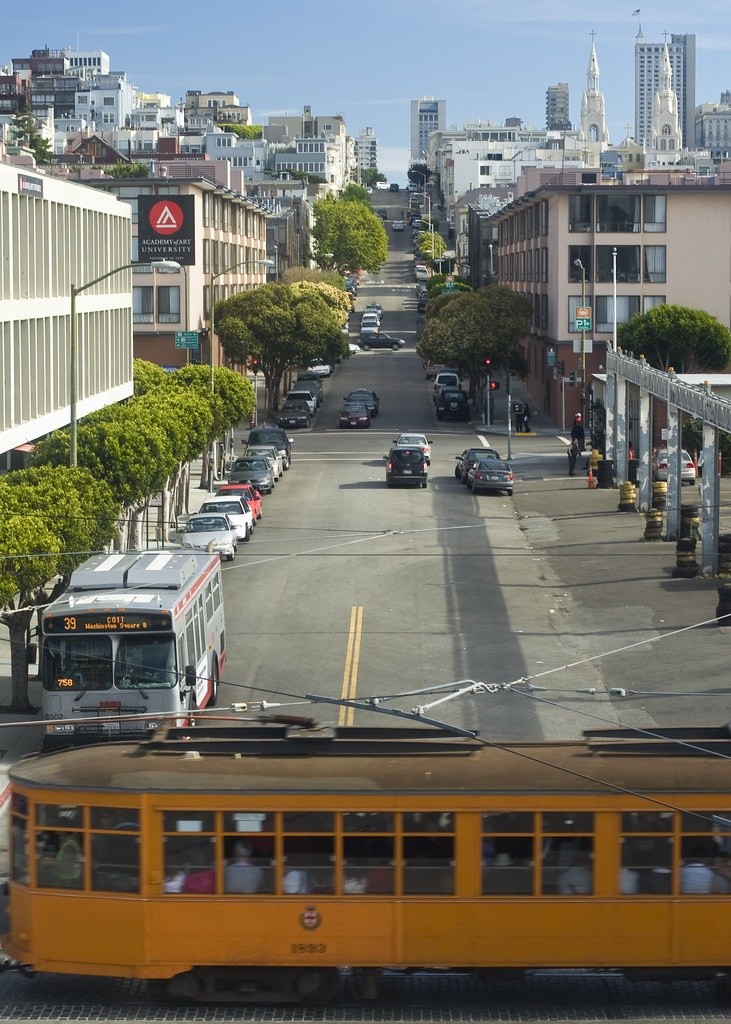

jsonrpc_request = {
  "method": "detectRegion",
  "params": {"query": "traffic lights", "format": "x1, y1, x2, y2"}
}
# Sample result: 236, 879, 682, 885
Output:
512, 402, 524, 415
482, 357, 492, 370
491, 381, 500, 391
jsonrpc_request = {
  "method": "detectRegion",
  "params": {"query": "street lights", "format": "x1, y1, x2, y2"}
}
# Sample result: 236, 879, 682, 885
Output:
67, 257, 179, 466
575, 259, 587, 451
210, 259, 275, 398
413, 218, 435, 261
413, 192, 431, 232
411, 169, 427, 215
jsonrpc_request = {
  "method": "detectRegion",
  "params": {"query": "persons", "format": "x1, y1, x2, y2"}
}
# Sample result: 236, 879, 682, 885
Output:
22, 811, 731, 896
567, 438, 579, 476
571, 413, 586, 451
516, 403, 532, 433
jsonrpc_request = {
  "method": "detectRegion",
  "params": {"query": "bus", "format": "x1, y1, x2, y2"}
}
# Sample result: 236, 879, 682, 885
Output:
39, 550, 227, 750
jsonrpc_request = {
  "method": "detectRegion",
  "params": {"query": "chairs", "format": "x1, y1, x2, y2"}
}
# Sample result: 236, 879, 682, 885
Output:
256, 463, 263, 467
487, 455, 494, 458
230, 505, 238, 510
239, 463, 247, 471
209, 505, 217, 512
193, 522, 205, 530
497, 465, 504, 469
214, 521, 222, 528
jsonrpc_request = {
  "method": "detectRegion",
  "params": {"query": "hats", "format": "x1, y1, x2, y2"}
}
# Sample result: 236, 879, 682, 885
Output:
494, 853, 513, 866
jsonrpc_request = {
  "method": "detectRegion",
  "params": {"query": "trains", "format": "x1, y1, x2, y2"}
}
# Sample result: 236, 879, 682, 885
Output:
2, 717, 731, 981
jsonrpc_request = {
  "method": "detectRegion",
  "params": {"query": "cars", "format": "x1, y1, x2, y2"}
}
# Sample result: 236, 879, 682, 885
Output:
292, 180, 472, 429
467, 458, 514, 496
180, 513, 238, 561
243, 445, 283, 482
197, 495, 253, 542
215, 484, 263, 526
392, 434, 434, 466
276, 398, 311, 427
454, 448, 501, 484
650, 449, 697, 485
228, 456, 275, 494
287, 390, 317, 417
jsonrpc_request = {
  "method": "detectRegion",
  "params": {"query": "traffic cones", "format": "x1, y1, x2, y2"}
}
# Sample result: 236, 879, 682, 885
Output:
585, 465, 596, 489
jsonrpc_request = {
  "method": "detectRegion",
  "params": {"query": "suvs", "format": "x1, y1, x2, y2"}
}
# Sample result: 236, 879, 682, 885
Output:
383, 446, 429, 488
241, 426, 291, 470
357, 332, 405, 351
